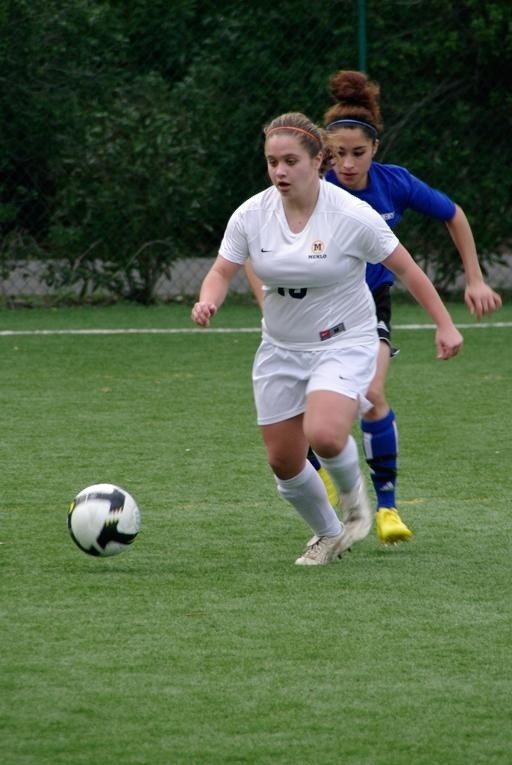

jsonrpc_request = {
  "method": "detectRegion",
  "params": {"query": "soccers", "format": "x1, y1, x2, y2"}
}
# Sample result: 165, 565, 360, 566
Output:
68, 483, 141, 557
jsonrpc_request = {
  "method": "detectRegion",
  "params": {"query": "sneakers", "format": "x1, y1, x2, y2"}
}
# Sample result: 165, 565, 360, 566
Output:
375, 506, 415, 543
295, 523, 353, 567
339, 489, 371, 541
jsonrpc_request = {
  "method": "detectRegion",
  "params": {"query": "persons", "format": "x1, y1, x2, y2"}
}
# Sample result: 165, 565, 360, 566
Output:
241, 68, 504, 549
188, 110, 466, 570
